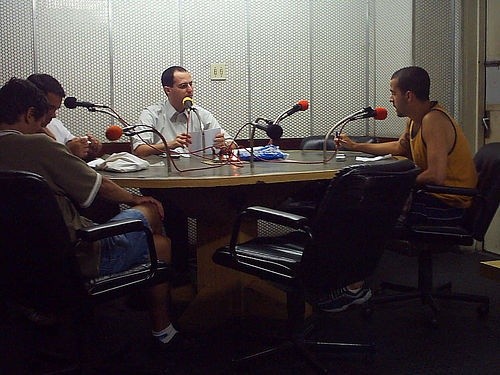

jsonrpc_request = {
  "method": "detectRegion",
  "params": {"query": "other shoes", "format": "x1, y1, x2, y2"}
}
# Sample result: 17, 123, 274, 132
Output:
153, 331, 197, 352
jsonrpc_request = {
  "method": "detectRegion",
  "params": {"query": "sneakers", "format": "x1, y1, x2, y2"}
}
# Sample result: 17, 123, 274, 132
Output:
316, 284, 373, 312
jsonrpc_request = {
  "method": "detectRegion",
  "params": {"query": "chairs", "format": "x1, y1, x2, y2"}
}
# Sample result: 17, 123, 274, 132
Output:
376, 142, 500, 325
212, 164, 421, 375
0, 170, 179, 375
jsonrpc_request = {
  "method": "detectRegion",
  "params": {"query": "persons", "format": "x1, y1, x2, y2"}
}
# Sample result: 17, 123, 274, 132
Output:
315, 66, 478, 313
131, 66, 238, 288
1, 73, 206, 351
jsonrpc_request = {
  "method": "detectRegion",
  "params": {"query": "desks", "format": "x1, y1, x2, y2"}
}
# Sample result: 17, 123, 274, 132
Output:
96, 150, 415, 188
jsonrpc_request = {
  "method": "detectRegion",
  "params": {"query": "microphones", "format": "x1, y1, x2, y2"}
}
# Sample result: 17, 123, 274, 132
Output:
105, 125, 138, 141
182, 97, 193, 108
279, 100, 308, 121
250, 122, 283, 139
64, 96, 108, 109
350, 107, 388, 121
